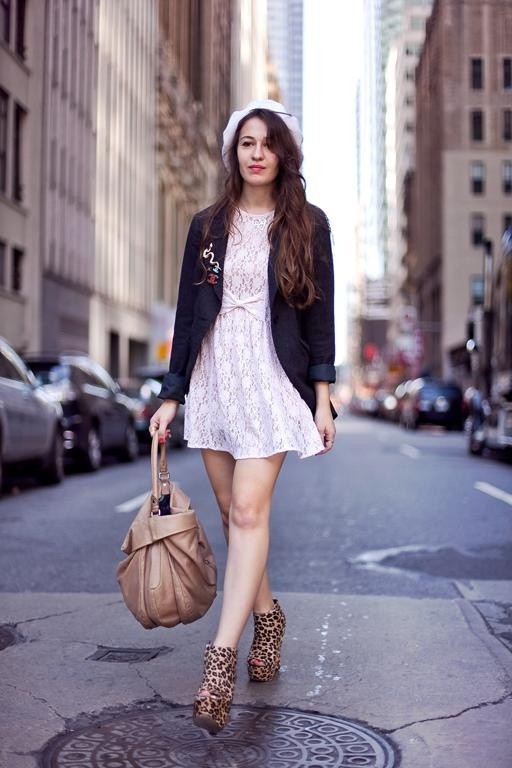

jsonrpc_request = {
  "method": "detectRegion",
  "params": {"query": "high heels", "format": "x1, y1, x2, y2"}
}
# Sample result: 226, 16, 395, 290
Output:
246, 599, 287, 682
192, 640, 239, 736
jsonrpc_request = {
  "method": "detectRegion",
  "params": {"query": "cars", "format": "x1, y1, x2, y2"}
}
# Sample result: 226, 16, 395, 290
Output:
0, 334, 184, 496
338, 375, 512, 460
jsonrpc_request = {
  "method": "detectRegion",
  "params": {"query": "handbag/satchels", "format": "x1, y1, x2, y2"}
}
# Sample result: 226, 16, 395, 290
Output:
115, 430, 218, 630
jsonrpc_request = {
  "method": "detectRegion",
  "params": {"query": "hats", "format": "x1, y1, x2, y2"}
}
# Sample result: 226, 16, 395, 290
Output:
221, 99, 304, 175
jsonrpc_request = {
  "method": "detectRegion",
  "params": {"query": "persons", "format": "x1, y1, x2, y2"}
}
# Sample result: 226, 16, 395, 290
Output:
144, 99, 340, 738
